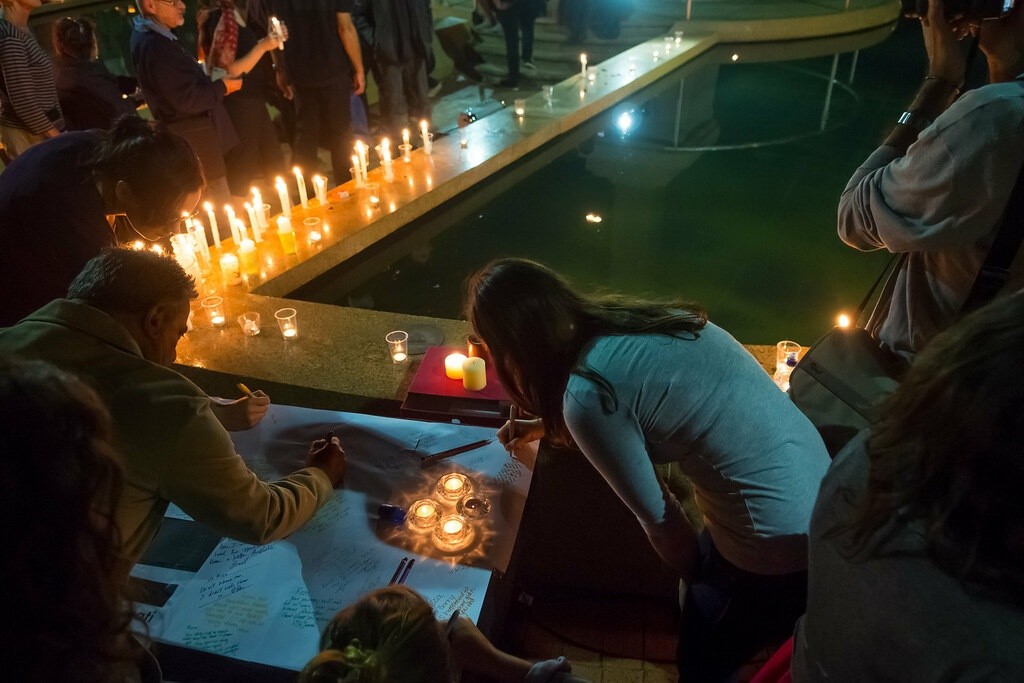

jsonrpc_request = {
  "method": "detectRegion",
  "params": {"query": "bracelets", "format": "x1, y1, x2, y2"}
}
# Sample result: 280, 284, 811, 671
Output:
897, 110, 932, 132
924, 75, 962, 95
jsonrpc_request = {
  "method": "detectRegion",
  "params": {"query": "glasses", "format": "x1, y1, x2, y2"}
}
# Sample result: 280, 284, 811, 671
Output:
117, 177, 199, 226
65, 15, 84, 34
155, 0, 177, 8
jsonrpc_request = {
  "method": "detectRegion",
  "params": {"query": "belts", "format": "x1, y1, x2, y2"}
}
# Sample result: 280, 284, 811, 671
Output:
167, 110, 212, 124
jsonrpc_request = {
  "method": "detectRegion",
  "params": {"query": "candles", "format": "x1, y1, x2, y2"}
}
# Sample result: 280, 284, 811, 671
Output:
419, 121, 434, 155
129, 165, 329, 339
458, 127, 469, 148
397, 125, 414, 164
675, 29, 684, 47
386, 329, 409, 363
775, 338, 804, 390
348, 141, 396, 206
410, 469, 489, 551
581, 54, 596, 80
513, 97, 526, 117
664, 35, 673, 52
445, 349, 487, 390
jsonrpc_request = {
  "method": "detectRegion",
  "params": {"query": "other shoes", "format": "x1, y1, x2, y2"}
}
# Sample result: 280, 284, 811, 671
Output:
500, 78, 518, 91
524, 57, 536, 70
426, 80, 442, 98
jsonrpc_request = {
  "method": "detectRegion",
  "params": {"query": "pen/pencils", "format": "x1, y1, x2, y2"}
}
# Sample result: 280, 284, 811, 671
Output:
398, 559, 416, 585
237, 383, 256, 397
388, 557, 407, 585
326, 430, 334, 442
421, 438, 495, 461
509, 405, 515, 457
445, 609, 460, 635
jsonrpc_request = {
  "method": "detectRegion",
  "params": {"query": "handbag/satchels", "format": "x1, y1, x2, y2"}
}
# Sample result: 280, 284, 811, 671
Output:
786, 324, 911, 459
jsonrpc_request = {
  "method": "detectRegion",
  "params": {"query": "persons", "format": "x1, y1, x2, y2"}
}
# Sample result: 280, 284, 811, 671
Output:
298, 584, 587, 683
0, 0, 59, 165
0, 113, 207, 329
790, 0, 1024, 458
49, 16, 138, 132
125, 0, 243, 201
0, 242, 347, 683
466, 256, 834, 683
490, 0, 548, 88
196, 0, 434, 205
790, 290, 1024, 683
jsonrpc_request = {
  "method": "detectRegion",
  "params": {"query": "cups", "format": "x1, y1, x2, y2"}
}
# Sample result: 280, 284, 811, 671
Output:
384, 329, 408, 364
542, 85, 553, 100
776, 340, 802, 372
303, 217, 323, 243
514, 99, 526, 118
365, 183, 380, 208
311, 176, 328, 200
263, 204, 271, 227
238, 311, 261, 337
200, 296, 227, 328
349, 166, 362, 186
273, 306, 298, 341
